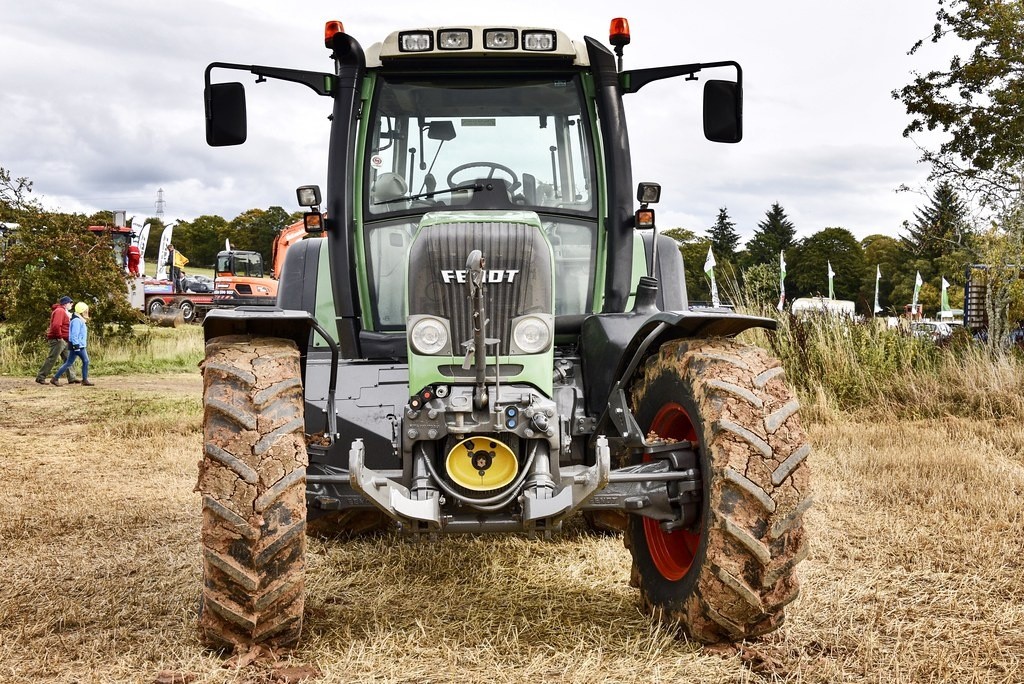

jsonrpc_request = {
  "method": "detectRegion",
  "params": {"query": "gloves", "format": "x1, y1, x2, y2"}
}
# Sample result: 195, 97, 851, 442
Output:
73, 344, 80, 349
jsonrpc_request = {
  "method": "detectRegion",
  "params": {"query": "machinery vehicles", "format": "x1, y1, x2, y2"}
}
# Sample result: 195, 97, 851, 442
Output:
195, 17, 817, 660
85, 209, 328, 328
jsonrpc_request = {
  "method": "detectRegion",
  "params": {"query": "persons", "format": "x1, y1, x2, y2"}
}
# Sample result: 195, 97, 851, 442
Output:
35, 296, 82, 384
180, 271, 189, 292
126, 246, 141, 277
163, 244, 189, 294
49, 302, 95, 387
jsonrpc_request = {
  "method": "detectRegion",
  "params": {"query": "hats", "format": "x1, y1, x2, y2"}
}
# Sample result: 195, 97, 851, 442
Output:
61, 296, 73, 304
75, 302, 88, 313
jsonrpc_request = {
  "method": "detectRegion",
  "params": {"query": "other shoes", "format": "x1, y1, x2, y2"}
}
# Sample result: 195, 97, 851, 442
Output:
36, 379, 49, 385
69, 379, 82, 384
81, 380, 94, 386
50, 377, 63, 387
